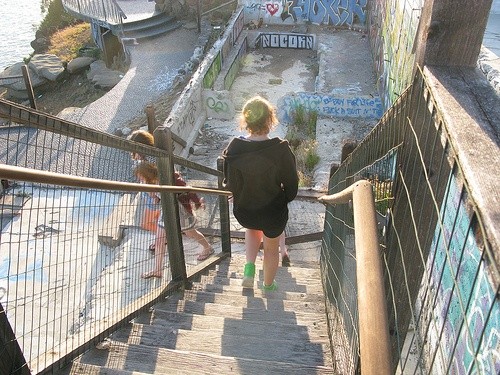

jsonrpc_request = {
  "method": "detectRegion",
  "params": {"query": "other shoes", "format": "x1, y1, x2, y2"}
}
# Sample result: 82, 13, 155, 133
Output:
149, 242, 168, 251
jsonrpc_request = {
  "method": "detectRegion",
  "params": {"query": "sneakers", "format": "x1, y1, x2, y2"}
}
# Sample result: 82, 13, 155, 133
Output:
281, 255, 290, 267
241, 263, 256, 288
262, 281, 279, 292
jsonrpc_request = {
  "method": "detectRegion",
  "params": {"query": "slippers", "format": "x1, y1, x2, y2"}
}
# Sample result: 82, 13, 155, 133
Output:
196, 249, 215, 261
141, 270, 164, 279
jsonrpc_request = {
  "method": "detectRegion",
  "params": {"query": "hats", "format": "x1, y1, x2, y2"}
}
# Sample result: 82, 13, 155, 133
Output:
242, 97, 271, 125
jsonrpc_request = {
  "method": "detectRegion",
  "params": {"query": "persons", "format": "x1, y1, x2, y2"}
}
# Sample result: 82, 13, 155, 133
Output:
134, 160, 215, 279
124, 131, 176, 252
222, 96, 299, 290
278, 231, 291, 267
